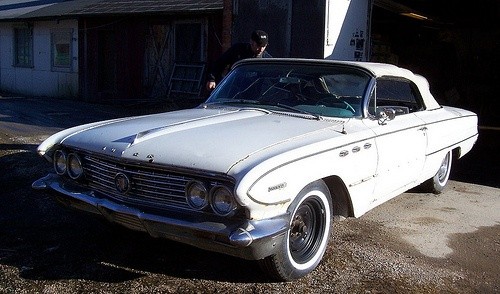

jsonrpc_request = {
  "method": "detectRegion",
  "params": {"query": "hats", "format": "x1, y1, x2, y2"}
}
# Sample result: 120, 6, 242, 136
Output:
251, 30, 269, 47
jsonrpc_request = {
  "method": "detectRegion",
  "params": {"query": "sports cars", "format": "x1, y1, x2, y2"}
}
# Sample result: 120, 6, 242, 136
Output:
31, 57, 479, 282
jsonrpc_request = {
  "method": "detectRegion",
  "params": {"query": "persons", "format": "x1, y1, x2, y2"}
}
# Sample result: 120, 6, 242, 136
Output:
202, 29, 275, 96
